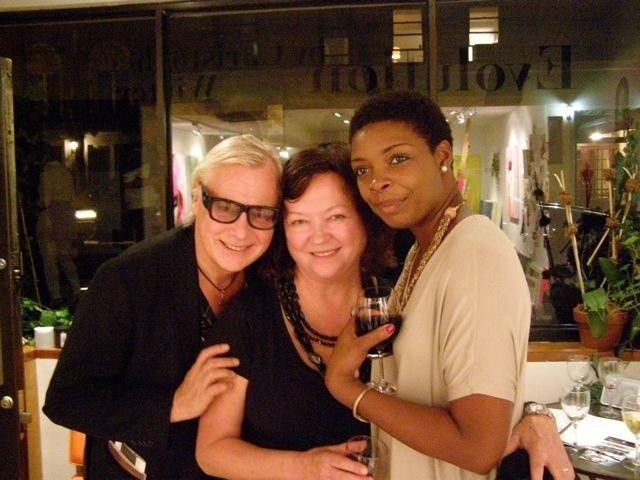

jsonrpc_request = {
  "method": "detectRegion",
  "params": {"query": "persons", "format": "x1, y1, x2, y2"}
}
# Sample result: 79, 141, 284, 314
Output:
324, 88, 532, 480
27, 142, 81, 309
194, 141, 576, 480
41, 134, 283, 480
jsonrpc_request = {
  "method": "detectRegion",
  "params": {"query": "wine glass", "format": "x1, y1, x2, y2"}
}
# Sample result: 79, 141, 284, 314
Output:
560, 386, 591, 456
353, 285, 402, 395
568, 354, 591, 409
619, 395, 640, 472
597, 356, 623, 420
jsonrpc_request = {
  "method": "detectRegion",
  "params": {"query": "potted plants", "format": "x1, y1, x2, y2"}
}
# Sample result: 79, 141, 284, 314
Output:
575, 109, 639, 349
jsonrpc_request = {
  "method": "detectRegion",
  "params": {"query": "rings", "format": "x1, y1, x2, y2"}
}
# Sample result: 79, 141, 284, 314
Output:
563, 468, 571, 472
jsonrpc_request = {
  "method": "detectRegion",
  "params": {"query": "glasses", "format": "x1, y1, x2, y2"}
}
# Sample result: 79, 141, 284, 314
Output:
202, 190, 282, 229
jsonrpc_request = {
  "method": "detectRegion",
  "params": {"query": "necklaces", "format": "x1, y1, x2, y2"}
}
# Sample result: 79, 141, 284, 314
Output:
281, 277, 340, 376
197, 265, 240, 302
392, 201, 465, 310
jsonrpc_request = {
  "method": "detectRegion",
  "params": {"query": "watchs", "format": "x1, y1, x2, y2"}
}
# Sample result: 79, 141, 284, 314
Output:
524, 401, 555, 420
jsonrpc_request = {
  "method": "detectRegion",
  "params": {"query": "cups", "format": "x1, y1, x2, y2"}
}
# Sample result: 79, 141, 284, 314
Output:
344, 433, 391, 480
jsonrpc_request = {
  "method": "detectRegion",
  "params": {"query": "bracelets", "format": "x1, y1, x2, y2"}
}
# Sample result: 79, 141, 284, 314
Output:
353, 386, 376, 424
